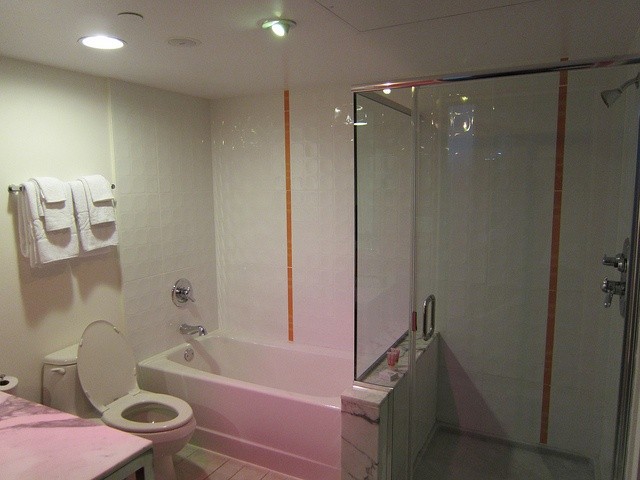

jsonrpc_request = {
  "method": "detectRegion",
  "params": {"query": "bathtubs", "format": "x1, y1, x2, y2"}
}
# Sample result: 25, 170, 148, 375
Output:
137, 329, 381, 479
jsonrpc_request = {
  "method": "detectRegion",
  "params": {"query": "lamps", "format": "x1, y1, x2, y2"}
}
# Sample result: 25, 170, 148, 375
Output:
269, 21, 293, 39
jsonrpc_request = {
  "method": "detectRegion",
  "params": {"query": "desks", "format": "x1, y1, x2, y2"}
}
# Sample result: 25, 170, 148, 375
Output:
0, 391, 154, 480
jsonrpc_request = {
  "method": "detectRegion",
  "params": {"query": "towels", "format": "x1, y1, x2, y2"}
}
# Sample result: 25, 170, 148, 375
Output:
33, 178, 74, 233
18, 181, 81, 270
84, 174, 117, 226
68, 181, 120, 260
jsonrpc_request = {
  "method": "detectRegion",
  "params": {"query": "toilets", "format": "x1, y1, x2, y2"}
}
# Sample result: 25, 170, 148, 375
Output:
42, 320, 197, 480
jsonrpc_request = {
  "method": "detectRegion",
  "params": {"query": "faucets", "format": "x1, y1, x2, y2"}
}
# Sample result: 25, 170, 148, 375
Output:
181, 323, 208, 338
